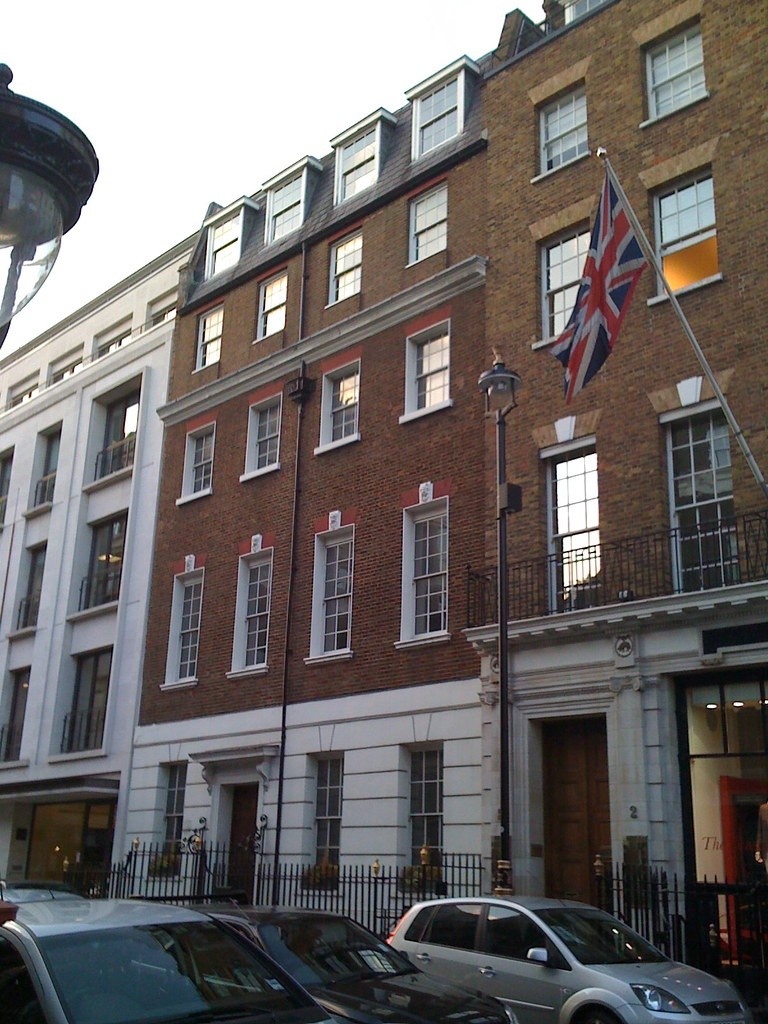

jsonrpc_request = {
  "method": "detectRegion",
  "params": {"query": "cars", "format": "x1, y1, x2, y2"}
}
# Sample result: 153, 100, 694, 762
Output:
384, 895, 756, 1024
166, 901, 520, 1024
0, 896, 337, 1024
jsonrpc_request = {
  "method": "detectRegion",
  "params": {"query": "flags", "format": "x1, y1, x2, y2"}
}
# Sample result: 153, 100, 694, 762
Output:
548, 164, 650, 406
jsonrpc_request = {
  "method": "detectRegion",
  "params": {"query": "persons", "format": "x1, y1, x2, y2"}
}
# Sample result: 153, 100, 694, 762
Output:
755, 801, 768, 874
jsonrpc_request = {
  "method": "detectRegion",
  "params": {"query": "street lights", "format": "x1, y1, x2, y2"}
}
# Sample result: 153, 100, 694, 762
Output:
477, 342, 525, 900
